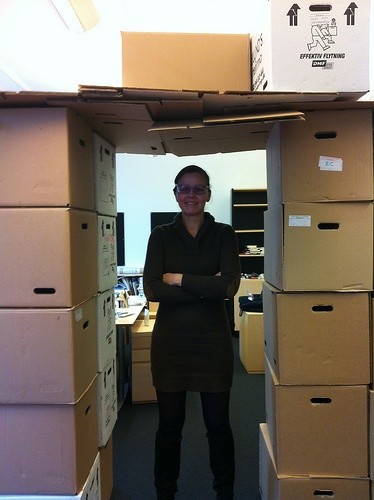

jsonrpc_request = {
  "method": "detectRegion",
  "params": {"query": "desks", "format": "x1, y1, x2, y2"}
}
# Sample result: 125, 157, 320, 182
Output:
131, 319, 157, 403
238, 308, 266, 374
115, 293, 148, 415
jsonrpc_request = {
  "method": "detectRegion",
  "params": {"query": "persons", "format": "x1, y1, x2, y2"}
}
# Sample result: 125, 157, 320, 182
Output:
142, 165, 241, 499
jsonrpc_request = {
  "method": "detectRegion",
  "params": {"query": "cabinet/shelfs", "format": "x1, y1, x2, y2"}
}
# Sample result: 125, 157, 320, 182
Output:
231, 188, 266, 331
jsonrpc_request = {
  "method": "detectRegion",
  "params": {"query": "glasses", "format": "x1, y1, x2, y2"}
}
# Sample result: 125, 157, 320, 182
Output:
176, 184, 210, 194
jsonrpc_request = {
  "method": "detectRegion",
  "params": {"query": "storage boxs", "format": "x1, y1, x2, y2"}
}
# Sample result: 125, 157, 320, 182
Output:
0, 0, 374, 500
